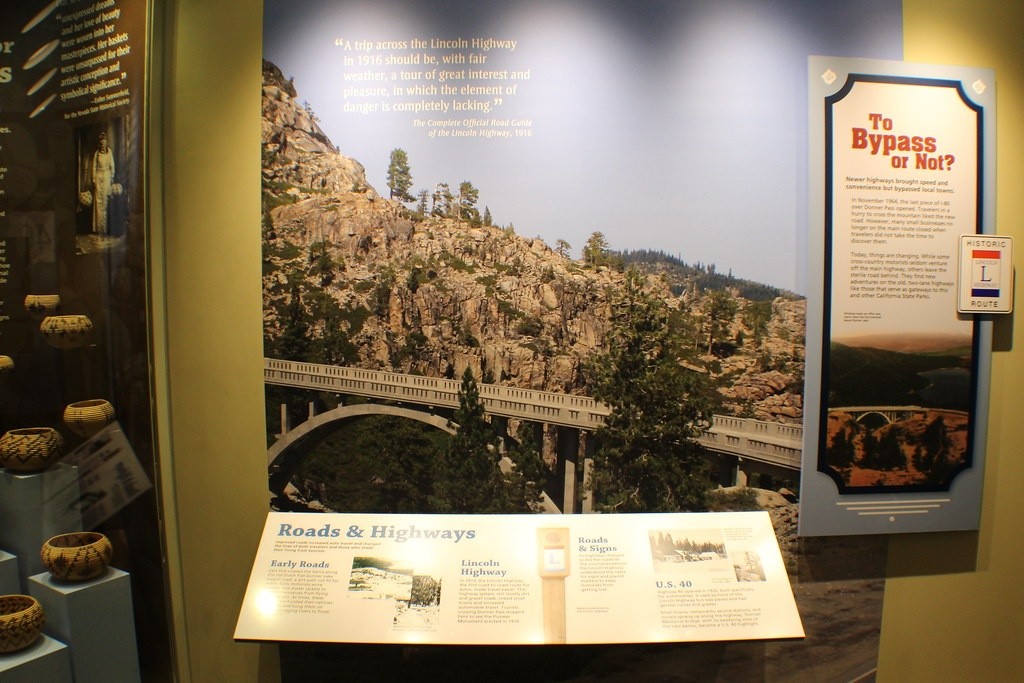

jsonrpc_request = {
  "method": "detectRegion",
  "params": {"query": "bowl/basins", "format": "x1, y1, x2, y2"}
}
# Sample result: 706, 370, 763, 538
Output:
25, 294, 60, 320
41, 532, 114, 583
0, 595, 44, 654
0, 427, 64, 473
63, 399, 116, 438
40, 314, 93, 351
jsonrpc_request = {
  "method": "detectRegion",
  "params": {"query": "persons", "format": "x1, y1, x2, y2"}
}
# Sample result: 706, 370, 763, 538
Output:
89, 130, 116, 232
